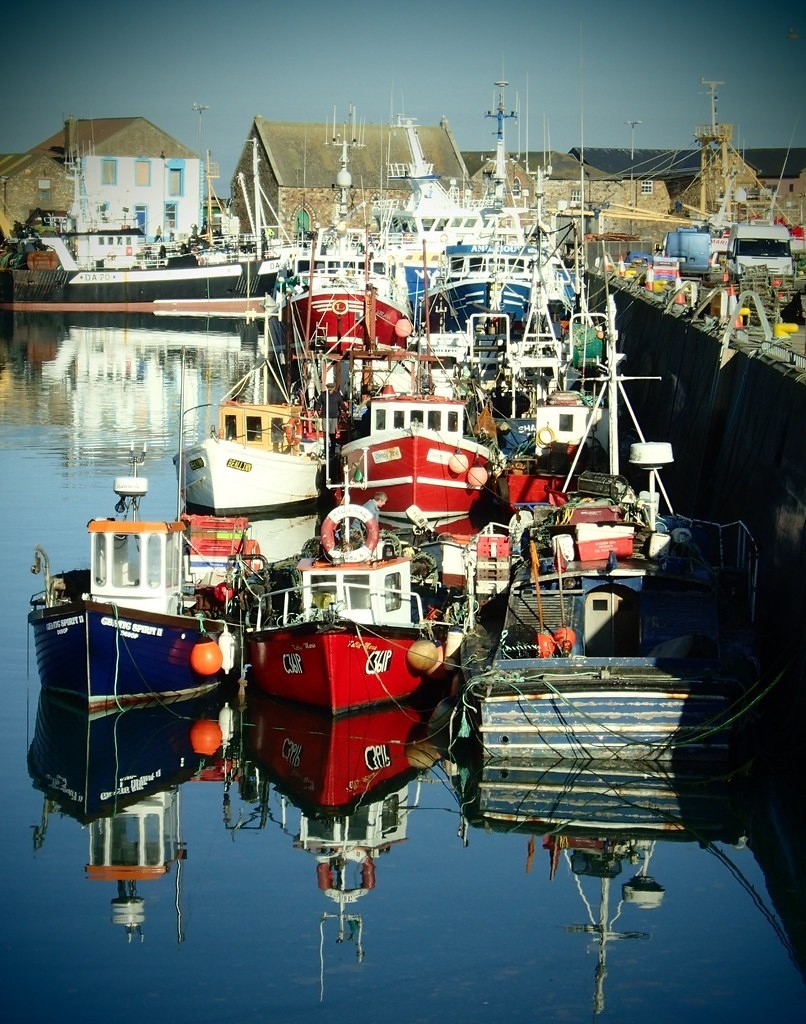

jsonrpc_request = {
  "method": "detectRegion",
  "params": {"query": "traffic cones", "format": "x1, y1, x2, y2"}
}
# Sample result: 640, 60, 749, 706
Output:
605, 255, 610, 272
645, 262, 655, 291
726, 284, 748, 330
616, 255, 627, 278
671, 268, 687, 305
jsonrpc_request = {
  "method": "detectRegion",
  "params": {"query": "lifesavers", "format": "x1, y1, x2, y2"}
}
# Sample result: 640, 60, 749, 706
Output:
286, 418, 303, 446
316, 847, 378, 904
322, 504, 380, 562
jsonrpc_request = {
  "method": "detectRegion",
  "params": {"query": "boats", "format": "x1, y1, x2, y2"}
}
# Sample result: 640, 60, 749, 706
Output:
0, 66, 673, 716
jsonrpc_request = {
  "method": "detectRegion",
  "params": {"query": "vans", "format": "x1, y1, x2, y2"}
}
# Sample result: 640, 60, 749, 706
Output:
725, 224, 800, 286
656, 227, 713, 282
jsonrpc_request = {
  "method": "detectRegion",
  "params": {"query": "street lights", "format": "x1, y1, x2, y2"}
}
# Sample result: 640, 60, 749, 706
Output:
623, 120, 643, 204
192, 103, 209, 158
